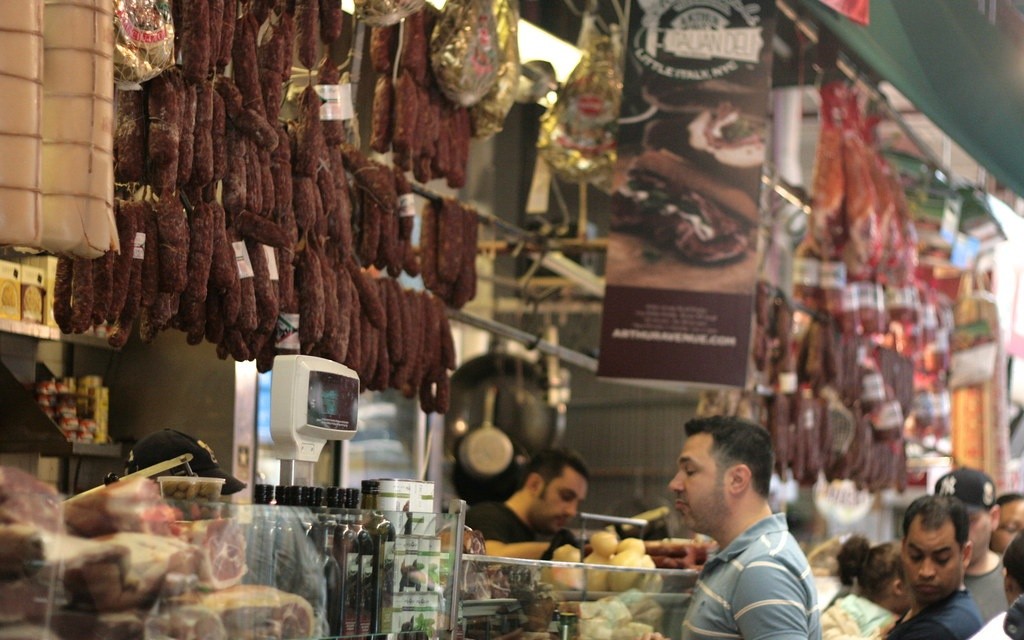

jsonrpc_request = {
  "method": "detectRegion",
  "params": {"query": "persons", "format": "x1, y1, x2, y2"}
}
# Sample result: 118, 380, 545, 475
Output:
820, 463, 1024, 640
435, 445, 593, 560
123, 426, 246, 495
668, 414, 819, 640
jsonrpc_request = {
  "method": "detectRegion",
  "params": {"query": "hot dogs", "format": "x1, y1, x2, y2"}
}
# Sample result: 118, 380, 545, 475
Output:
607, 150, 759, 263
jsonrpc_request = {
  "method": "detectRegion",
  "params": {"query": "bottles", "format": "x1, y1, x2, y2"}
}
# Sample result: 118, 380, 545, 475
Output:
255, 477, 393, 640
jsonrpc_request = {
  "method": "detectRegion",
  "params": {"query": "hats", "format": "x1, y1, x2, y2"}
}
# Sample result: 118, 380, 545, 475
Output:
934, 467, 996, 513
124, 428, 246, 495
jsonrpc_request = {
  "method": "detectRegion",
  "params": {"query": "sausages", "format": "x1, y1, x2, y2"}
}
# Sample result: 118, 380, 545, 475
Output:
53, 0, 477, 413
752, 279, 915, 491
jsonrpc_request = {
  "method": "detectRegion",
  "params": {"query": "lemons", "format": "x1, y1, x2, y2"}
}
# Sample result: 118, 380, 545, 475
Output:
553, 532, 661, 594
